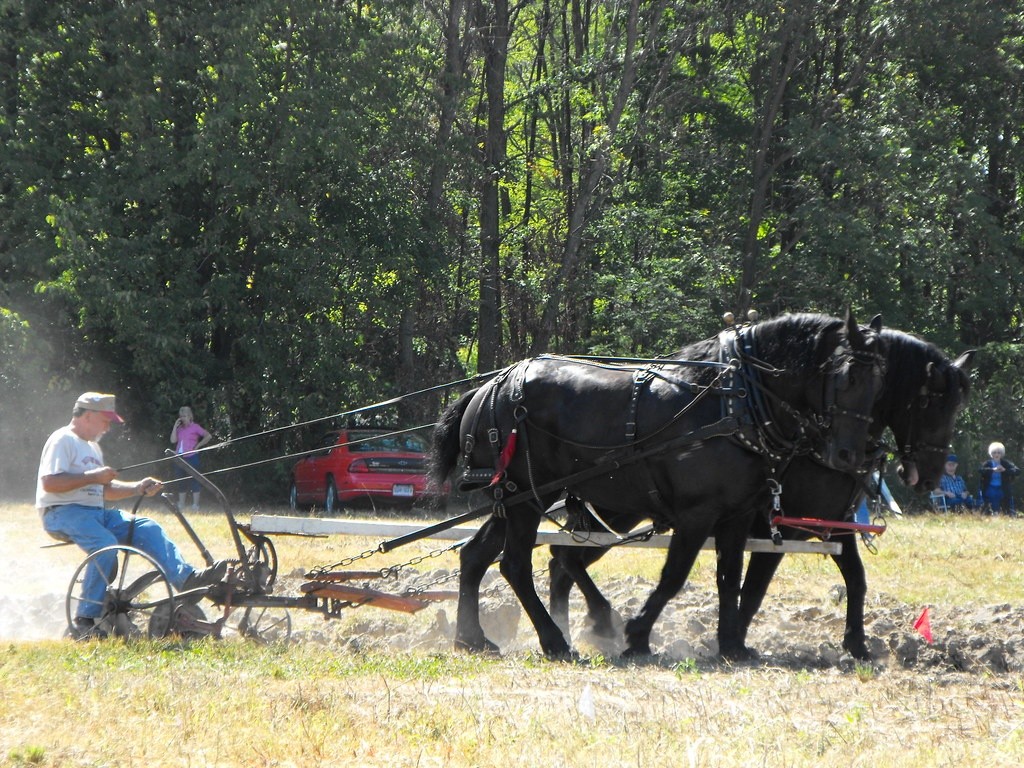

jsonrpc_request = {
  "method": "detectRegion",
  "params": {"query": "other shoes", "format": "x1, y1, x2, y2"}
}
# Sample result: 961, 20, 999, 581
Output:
72, 616, 106, 635
182, 560, 227, 605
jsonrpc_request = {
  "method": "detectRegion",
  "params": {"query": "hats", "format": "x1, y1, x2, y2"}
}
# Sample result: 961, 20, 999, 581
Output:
75, 392, 125, 424
947, 454, 959, 464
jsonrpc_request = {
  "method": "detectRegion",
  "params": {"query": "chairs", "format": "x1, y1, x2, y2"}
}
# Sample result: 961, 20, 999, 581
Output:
929, 491, 973, 516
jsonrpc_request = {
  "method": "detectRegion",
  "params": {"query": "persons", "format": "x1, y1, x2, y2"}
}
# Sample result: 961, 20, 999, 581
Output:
36, 392, 228, 638
933, 454, 974, 512
170, 406, 211, 511
978, 442, 1022, 515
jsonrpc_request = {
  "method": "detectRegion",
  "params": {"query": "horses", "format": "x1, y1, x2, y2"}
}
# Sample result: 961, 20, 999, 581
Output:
547, 315, 979, 663
425, 303, 890, 663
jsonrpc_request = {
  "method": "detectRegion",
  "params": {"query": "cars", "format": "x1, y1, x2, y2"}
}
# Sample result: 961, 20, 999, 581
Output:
287, 428, 453, 520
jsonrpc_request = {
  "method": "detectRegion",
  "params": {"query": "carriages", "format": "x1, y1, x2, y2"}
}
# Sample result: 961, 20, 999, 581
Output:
45, 308, 979, 664
